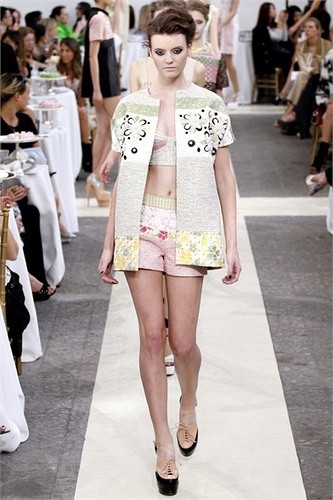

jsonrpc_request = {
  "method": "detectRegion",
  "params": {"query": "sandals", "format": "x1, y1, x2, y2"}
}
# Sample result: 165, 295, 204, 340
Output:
32, 281, 56, 296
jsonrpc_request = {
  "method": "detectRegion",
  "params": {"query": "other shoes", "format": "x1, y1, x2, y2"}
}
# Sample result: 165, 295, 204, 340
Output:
61, 231, 77, 238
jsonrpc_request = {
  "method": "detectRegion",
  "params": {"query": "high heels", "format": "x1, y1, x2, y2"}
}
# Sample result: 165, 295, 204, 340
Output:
153, 433, 179, 496
87, 173, 110, 200
227, 91, 243, 110
177, 394, 198, 456
277, 112, 295, 133
306, 174, 326, 195
85, 185, 109, 207
163, 353, 177, 375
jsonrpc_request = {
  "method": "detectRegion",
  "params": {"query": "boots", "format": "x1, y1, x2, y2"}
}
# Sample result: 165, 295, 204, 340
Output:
309, 140, 330, 174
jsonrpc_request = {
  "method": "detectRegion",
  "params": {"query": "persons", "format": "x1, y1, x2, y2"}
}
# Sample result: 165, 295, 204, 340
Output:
0, 0, 333, 375
97, 6, 243, 496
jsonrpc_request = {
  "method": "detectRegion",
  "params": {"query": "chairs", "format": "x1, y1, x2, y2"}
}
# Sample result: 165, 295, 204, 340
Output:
255, 63, 282, 100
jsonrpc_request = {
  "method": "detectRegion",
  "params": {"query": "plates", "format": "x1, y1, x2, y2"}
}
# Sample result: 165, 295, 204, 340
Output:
25, 103, 63, 111
31, 75, 68, 81
0, 135, 41, 144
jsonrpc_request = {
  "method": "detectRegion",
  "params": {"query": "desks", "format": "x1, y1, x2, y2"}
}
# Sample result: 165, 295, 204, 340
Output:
0, 42, 95, 452
